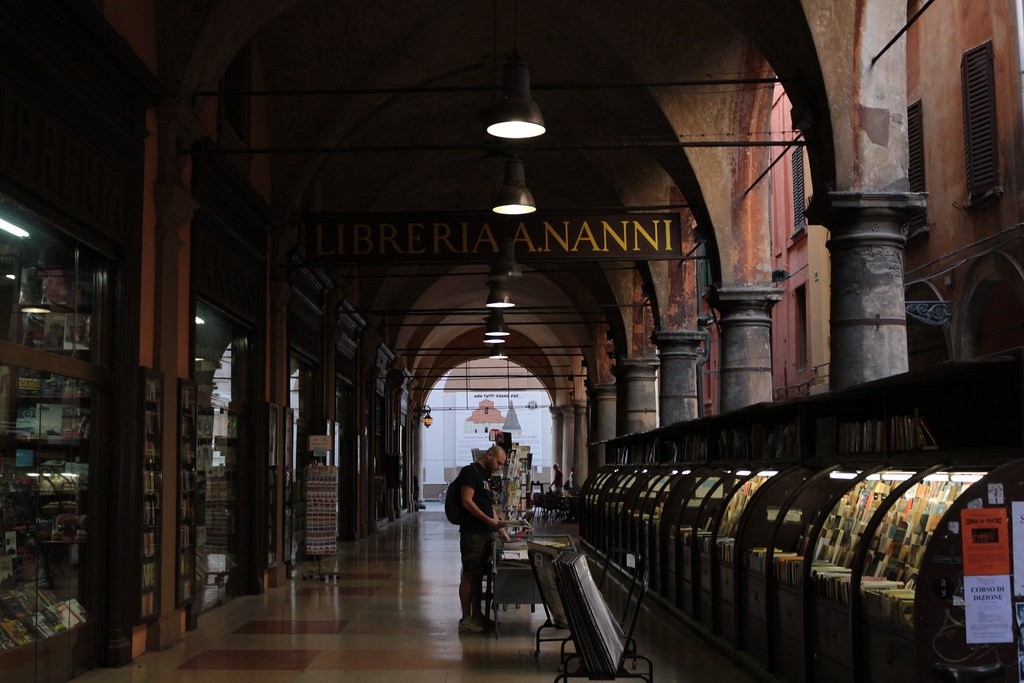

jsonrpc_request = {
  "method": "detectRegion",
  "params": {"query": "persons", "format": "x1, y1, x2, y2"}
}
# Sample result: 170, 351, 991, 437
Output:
568, 467, 574, 490
548, 463, 563, 492
457, 446, 512, 634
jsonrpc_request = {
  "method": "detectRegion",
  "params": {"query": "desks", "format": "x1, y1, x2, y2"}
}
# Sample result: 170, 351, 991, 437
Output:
551, 495, 579, 523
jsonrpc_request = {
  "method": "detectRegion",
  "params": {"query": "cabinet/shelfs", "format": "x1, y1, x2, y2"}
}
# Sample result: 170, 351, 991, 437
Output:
140, 359, 249, 630
577, 352, 1024, 683
0, 185, 108, 683
482, 534, 578, 639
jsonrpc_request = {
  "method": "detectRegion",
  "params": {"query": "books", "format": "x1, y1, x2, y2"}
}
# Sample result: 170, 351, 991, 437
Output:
0, 260, 239, 647
472, 444, 531, 565
582, 470, 986, 631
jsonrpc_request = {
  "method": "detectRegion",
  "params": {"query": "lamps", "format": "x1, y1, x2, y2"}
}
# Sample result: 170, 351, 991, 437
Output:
485, 282, 516, 308
0, 219, 30, 239
482, 315, 506, 344
486, 238, 523, 279
488, 343, 509, 360
481, 0, 547, 140
484, 307, 511, 337
492, 149, 537, 217
421, 405, 432, 428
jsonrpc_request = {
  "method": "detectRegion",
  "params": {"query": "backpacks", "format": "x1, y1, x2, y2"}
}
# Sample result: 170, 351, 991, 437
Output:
445, 463, 479, 525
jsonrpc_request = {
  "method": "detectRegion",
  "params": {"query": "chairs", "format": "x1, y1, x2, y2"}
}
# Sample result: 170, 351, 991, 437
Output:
533, 492, 549, 521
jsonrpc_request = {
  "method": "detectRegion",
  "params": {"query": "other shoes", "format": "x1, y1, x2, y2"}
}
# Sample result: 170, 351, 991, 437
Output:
472, 614, 499, 627
459, 619, 485, 632
